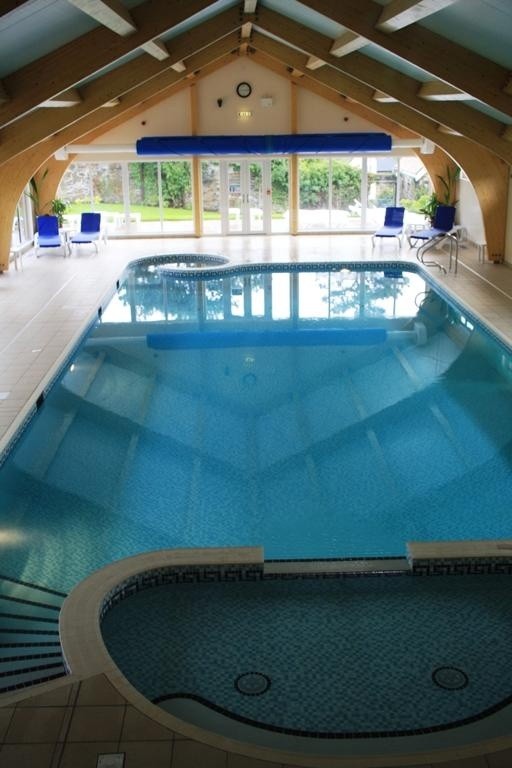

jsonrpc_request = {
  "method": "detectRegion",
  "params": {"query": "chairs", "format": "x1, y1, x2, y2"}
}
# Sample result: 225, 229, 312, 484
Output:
410, 203, 462, 254
34, 214, 66, 258
68, 211, 105, 257
372, 203, 408, 249
